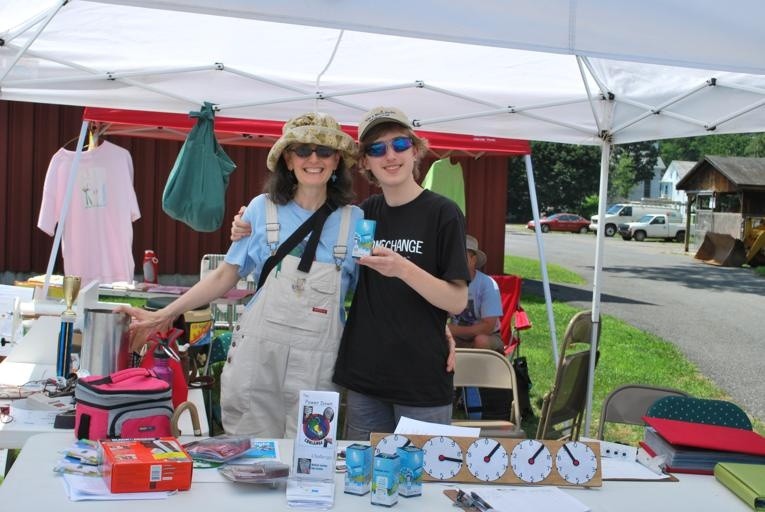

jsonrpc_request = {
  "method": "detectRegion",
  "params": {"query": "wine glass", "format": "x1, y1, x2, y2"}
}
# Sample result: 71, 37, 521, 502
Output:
60, 276, 82, 315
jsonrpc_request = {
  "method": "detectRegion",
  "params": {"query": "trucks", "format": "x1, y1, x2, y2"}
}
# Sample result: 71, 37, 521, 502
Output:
590, 201, 680, 236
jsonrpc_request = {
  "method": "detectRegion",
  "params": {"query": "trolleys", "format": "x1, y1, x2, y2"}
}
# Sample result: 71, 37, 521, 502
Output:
75, 330, 200, 437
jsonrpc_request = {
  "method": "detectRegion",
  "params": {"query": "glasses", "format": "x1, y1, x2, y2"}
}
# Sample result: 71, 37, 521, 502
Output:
286, 145, 339, 158
364, 137, 413, 156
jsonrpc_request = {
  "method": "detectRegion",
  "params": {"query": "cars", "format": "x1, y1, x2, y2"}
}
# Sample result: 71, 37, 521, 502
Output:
528, 212, 591, 235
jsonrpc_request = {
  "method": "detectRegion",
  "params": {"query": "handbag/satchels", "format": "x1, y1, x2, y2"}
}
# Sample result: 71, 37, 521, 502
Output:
139, 317, 187, 408
479, 356, 534, 420
75, 368, 174, 441
161, 101, 236, 233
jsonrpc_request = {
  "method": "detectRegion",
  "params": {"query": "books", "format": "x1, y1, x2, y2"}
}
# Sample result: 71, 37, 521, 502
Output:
712, 461, 762, 512
639, 415, 765, 479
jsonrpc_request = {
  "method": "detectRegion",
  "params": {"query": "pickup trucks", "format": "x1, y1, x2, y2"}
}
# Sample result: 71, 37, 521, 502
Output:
617, 214, 685, 241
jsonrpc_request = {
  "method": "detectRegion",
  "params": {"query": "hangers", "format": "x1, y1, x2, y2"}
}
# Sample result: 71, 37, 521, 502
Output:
61, 127, 104, 152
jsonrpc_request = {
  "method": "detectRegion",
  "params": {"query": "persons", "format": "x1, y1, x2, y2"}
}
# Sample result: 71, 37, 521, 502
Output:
113, 114, 368, 440
229, 109, 470, 439
446, 234, 505, 355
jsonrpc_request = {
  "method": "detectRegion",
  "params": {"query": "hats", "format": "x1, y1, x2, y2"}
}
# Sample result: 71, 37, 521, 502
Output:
358, 106, 413, 141
466, 235, 487, 268
266, 113, 360, 173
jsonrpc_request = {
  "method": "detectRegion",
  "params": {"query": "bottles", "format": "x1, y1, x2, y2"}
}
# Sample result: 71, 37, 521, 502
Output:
143, 249, 159, 283
